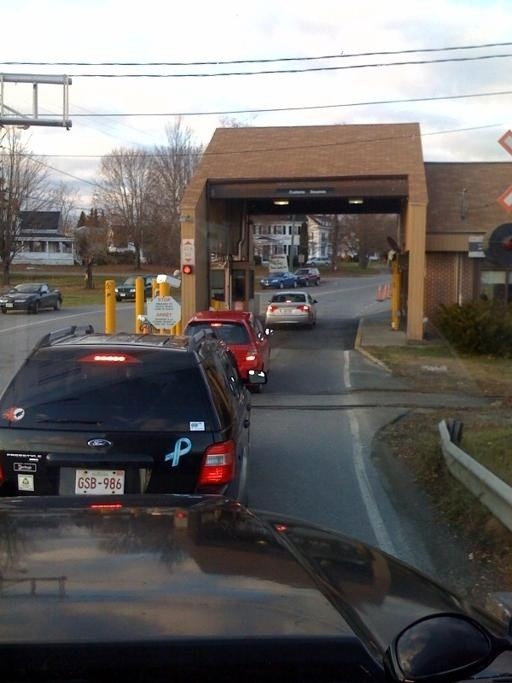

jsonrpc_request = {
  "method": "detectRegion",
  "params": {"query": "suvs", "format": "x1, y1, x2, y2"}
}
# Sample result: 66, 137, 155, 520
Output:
0, 316, 255, 506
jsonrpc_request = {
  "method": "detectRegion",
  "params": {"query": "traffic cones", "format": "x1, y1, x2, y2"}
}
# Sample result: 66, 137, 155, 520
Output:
375, 282, 392, 302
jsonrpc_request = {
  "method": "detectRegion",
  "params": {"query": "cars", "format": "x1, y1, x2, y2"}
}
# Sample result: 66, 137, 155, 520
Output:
0, 489, 512, 682
350, 251, 379, 263
114, 253, 332, 396
0, 279, 64, 316
